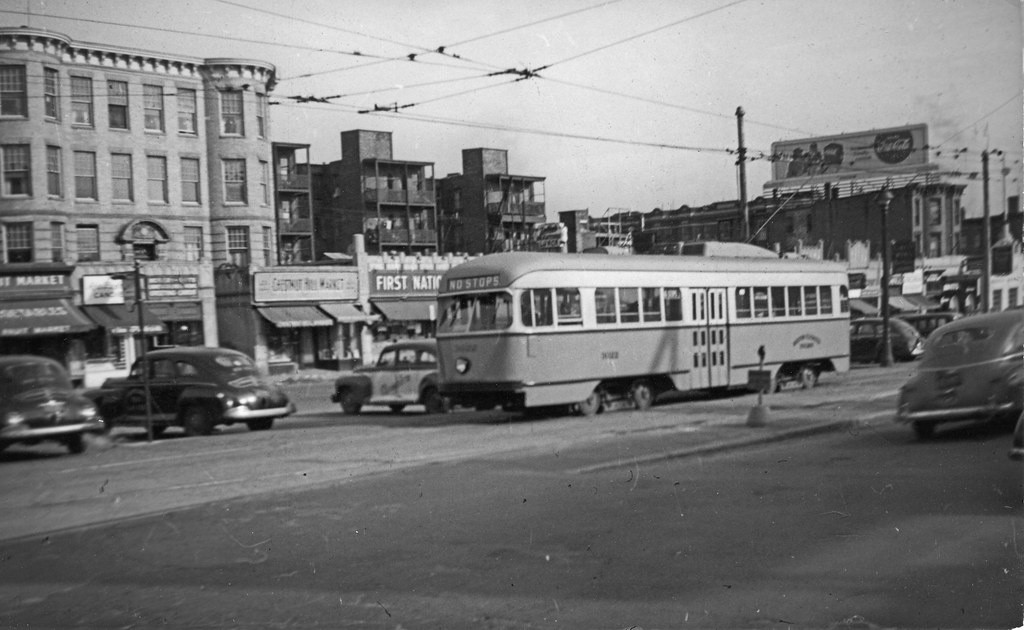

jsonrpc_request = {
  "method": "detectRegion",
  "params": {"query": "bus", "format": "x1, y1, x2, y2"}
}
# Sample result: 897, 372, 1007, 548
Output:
434, 241, 852, 416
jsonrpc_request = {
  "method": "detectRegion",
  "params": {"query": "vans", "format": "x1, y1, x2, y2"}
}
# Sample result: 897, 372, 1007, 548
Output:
881, 313, 962, 340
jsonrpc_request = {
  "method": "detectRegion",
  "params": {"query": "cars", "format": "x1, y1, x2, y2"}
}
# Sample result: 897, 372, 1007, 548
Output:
898, 309, 1024, 440
0, 355, 103, 457
326, 339, 452, 414
76, 344, 296, 438
849, 317, 927, 364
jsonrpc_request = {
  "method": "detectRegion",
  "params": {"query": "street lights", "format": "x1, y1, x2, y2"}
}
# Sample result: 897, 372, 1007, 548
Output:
874, 189, 894, 364
